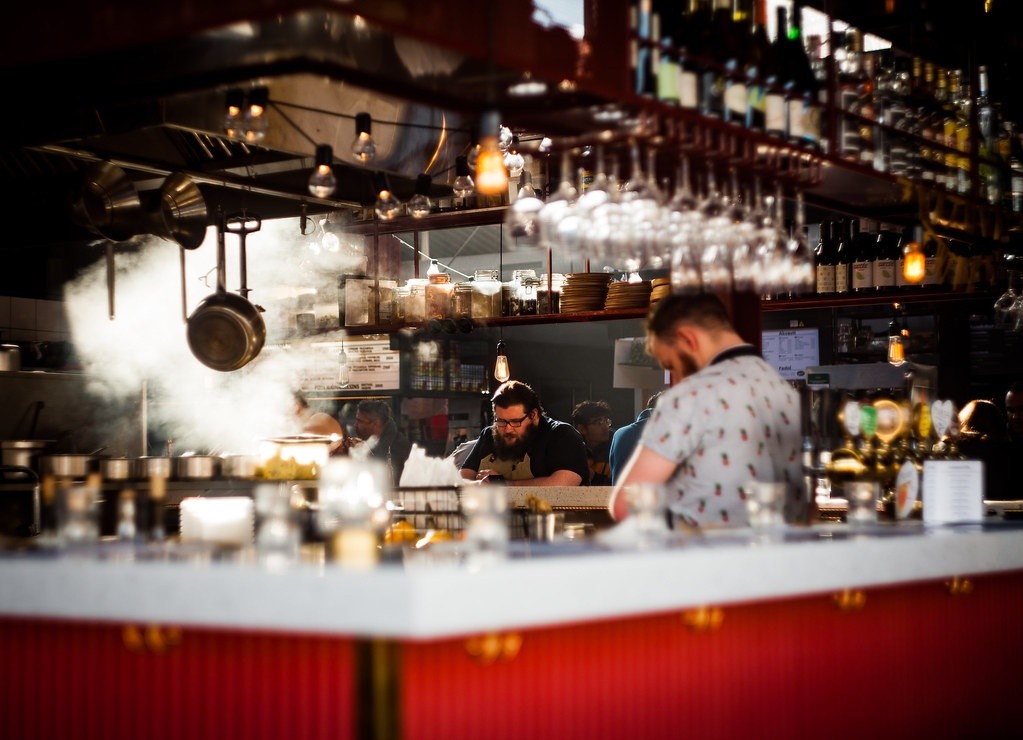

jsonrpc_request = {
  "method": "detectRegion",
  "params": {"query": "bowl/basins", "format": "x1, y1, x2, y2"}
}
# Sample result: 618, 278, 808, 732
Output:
0, 439, 44, 480
99, 457, 140, 481
51, 453, 93, 478
137, 456, 175, 481
222, 454, 256, 481
177, 455, 220, 480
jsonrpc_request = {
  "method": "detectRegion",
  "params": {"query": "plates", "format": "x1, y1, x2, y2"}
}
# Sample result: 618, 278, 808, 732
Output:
559, 270, 652, 313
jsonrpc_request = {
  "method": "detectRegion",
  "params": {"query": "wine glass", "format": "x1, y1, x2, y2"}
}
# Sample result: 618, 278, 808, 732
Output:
498, 110, 815, 299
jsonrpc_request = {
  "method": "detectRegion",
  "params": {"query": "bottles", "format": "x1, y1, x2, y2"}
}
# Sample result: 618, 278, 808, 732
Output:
427, 259, 439, 276
652, 0, 1023, 214
784, 218, 945, 298
367, 269, 565, 324
887, 335, 904, 363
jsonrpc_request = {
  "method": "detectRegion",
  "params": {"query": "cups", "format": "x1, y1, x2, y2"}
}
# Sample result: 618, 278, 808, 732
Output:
527, 512, 564, 546
842, 479, 879, 533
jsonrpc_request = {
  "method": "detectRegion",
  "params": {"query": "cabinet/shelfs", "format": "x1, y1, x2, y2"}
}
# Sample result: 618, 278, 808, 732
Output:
0, 0, 1023, 431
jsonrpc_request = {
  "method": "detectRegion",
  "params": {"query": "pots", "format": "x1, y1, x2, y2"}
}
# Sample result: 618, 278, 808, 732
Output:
186, 224, 267, 372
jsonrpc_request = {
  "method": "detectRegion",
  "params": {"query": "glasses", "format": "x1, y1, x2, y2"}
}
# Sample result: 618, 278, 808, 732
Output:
494, 414, 529, 427
583, 419, 611, 427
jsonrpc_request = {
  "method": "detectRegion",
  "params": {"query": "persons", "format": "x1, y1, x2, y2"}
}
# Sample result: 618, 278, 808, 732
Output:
610, 285, 804, 528
932, 383, 1023, 501
354, 399, 414, 483
573, 399, 617, 486
458, 380, 591, 487
609, 391, 664, 486
